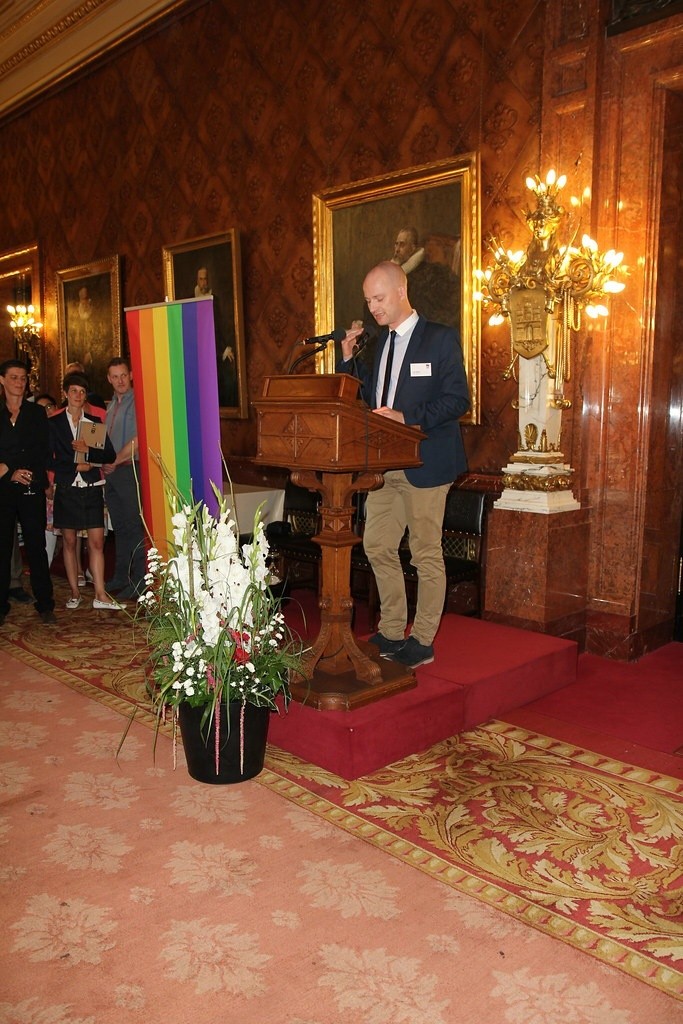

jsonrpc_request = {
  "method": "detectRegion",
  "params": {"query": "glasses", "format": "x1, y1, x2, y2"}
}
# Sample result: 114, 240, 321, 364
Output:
44, 403, 56, 411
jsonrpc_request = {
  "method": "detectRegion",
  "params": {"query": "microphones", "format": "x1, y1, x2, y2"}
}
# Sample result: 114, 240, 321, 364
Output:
297, 328, 346, 345
351, 323, 378, 356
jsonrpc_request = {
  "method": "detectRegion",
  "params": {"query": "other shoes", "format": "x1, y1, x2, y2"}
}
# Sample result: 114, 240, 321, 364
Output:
93, 598, 127, 610
40, 610, 58, 624
85, 569, 94, 583
116, 582, 148, 599
8, 586, 33, 604
0, 614, 7, 626
104, 580, 130, 592
66, 594, 83, 609
77, 571, 86, 586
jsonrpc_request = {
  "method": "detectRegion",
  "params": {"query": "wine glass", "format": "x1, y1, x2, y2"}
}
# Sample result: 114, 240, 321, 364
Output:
23, 473, 35, 495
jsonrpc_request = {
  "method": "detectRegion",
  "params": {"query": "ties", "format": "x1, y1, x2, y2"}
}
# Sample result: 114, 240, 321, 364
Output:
381, 330, 396, 407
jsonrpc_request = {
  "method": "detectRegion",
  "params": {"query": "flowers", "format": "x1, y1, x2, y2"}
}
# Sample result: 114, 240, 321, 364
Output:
133, 482, 311, 775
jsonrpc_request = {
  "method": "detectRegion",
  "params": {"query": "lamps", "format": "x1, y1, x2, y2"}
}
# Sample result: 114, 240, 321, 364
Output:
475, 171, 626, 330
7, 305, 43, 392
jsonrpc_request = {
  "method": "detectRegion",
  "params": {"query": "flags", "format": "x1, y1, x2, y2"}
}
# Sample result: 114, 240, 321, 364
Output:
123, 295, 222, 600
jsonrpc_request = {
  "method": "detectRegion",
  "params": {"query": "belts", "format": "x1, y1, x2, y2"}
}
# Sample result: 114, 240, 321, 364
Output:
72, 480, 107, 487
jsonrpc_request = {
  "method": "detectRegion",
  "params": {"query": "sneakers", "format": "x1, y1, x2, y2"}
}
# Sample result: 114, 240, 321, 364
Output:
382, 635, 435, 669
367, 633, 408, 657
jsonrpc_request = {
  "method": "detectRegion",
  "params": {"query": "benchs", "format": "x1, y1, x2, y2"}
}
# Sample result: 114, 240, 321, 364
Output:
266, 485, 489, 617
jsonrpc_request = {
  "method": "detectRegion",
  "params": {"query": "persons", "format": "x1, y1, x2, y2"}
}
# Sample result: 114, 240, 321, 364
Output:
492, 192, 590, 316
0, 359, 147, 621
339, 260, 470, 669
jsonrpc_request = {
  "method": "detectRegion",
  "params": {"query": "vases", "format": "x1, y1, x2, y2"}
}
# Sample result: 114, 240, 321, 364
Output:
179, 699, 270, 786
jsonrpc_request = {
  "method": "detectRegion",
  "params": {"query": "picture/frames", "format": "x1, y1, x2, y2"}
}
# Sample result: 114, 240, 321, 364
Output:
161, 228, 248, 421
53, 254, 122, 402
312, 155, 483, 428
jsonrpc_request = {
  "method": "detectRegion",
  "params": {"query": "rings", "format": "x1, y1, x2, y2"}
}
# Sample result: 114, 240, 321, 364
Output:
21, 473, 23, 477
